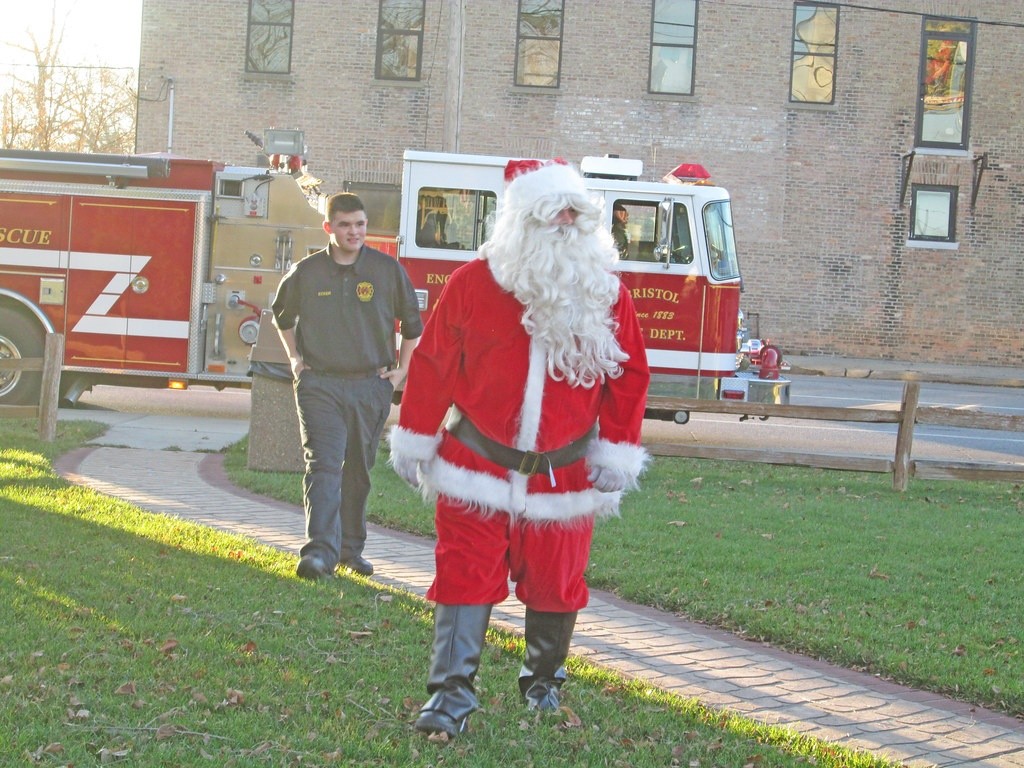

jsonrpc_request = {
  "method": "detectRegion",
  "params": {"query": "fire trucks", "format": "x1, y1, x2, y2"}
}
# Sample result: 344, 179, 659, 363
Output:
0, 126, 793, 425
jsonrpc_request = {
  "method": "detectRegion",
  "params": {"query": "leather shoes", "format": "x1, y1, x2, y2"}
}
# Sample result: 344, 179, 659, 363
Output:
340, 554, 373, 575
296, 555, 329, 580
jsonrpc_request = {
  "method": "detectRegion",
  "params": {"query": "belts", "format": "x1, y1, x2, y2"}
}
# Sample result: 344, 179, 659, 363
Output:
313, 366, 389, 381
445, 407, 602, 476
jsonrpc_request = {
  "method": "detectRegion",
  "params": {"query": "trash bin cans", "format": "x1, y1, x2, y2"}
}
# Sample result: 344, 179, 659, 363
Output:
247, 308, 306, 472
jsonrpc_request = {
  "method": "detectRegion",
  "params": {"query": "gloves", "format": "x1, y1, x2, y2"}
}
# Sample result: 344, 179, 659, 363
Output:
587, 463, 630, 492
392, 453, 430, 489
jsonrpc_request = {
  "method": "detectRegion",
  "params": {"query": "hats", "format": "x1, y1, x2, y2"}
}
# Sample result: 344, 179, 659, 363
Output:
504, 157, 586, 211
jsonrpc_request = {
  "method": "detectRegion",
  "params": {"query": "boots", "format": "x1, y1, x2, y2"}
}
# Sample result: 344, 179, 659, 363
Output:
415, 602, 494, 736
517, 607, 579, 714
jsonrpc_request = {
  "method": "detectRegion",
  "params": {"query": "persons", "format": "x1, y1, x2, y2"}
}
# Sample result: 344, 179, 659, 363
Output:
391, 161, 650, 740
269, 192, 423, 583
612, 204, 629, 259
418, 197, 465, 249
